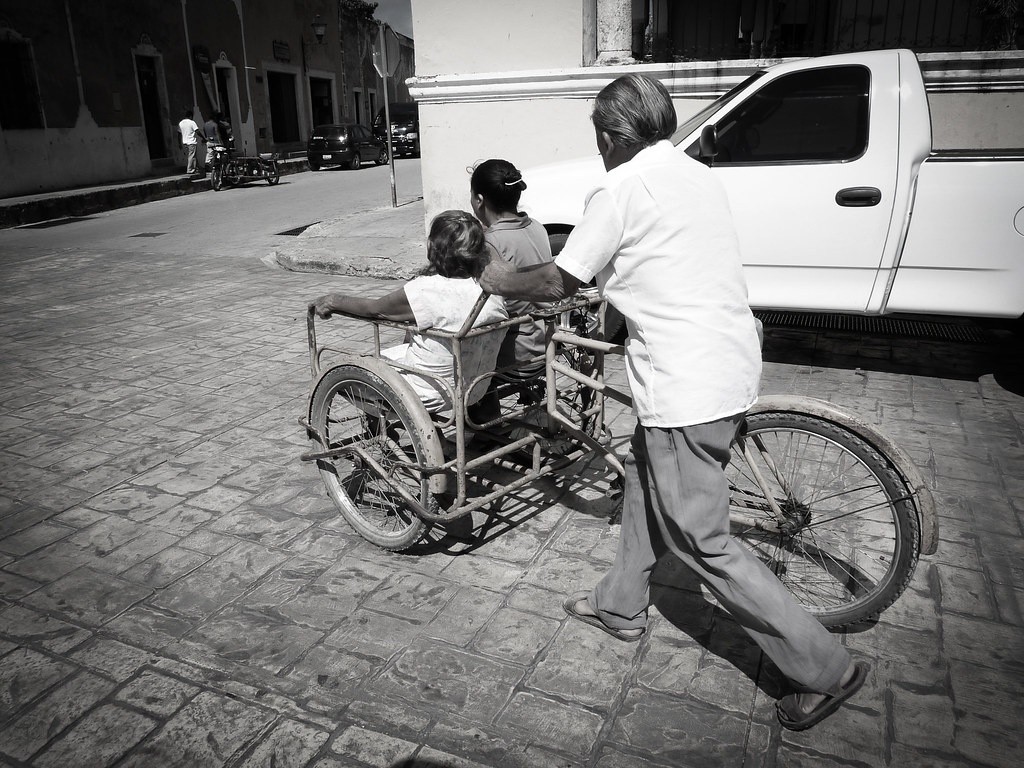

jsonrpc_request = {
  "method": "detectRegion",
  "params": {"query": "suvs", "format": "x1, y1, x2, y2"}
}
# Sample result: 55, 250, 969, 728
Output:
373, 101, 420, 158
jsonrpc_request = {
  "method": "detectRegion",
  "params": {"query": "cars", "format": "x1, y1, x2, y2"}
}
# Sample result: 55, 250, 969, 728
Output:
307, 123, 389, 172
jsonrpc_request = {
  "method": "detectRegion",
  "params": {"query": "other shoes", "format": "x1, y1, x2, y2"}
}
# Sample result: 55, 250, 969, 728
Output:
464, 414, 502, 431
187, 170, 198, 174
204, 163, 211, 172
367, 432, 399, 441
405, 439, 454, 459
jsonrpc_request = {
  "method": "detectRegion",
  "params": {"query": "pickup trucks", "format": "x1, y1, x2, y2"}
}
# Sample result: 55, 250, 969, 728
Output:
659, 46, 1024, 319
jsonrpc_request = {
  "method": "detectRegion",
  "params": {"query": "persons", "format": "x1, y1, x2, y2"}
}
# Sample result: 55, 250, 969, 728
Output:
176, 110, 207, 174
203, 112, 236, 172
479, 72, 869, 732
470, 157, 557, 442
303, 207, 508, 469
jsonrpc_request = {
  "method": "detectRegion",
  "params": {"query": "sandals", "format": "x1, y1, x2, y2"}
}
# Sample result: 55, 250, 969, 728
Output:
562, 589, 641, 642
778, 662, 865, 731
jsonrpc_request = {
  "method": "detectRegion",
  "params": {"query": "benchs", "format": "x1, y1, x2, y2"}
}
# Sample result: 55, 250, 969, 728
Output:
304, 297, 607, 428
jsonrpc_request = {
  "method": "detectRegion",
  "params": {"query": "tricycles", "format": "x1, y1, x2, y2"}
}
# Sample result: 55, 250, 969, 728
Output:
204, 134, 281, 191
296, 239, 938, 631
